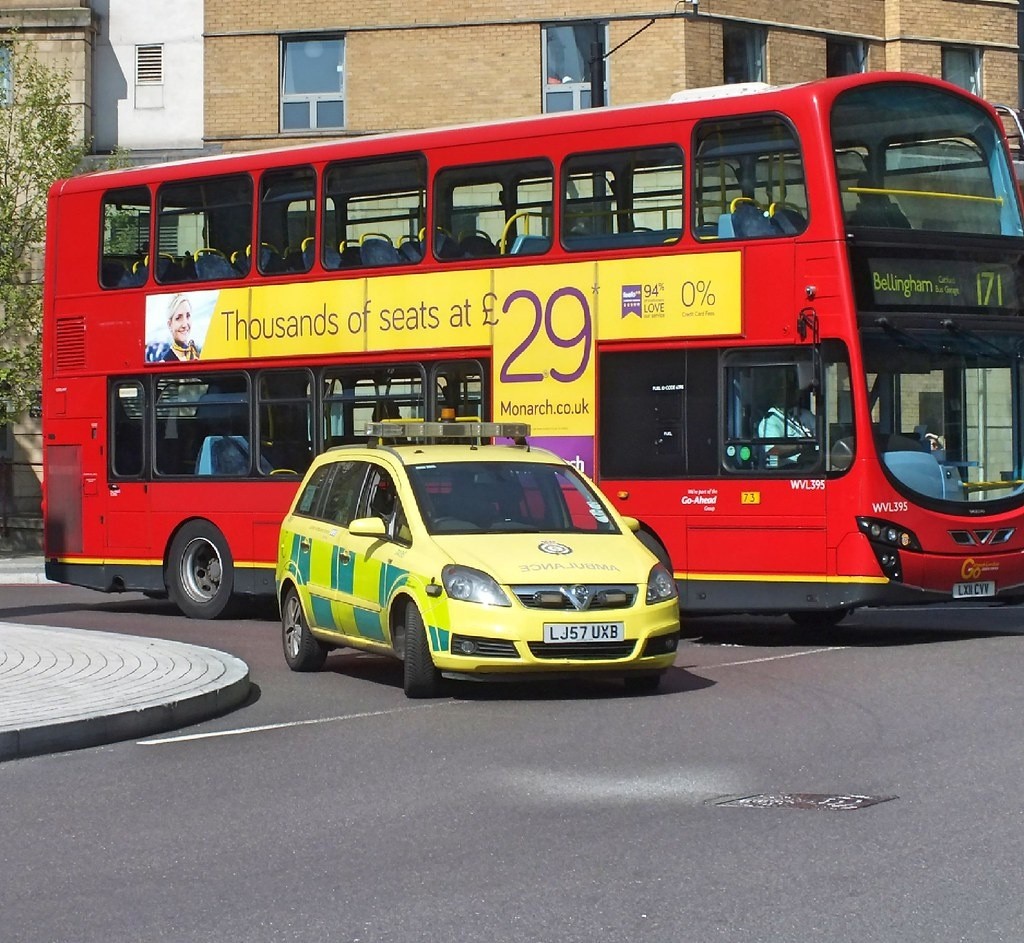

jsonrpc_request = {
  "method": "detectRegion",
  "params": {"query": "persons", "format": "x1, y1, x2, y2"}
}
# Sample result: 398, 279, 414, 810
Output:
757, 367, 816, 468
161, 294, 199, 362
846, 178, 912, 229
925, 433, 946, 450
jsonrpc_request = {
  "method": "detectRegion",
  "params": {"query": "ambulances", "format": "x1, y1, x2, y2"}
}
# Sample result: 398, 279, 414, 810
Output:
275, 407, 680, 698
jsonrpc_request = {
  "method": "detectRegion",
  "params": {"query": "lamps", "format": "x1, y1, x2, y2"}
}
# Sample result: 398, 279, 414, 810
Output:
25, 382, 42, 419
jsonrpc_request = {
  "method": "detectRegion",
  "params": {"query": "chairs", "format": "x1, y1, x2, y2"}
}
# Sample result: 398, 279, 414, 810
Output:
418, 226, 456, 260
731, 197, 783, 238
466, 473, 507, 529
340, 238, 363, 267
284, 246, 305, 274
246, 243, 285, 275
194, 248, 238, 280
132, 261, 145, 286
231, 250, 250, 277
300, 237, 340, 271
495, 237, 520, 256
768, 201, 805, 236
359, 232, 401, 267
182, 254, 197, 281
398, 234, 422, 262
458, 228, 496, 260
144, 252, 179, 283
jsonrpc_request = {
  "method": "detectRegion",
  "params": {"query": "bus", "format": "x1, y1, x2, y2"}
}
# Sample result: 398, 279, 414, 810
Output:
40, 67, 1023, 638
